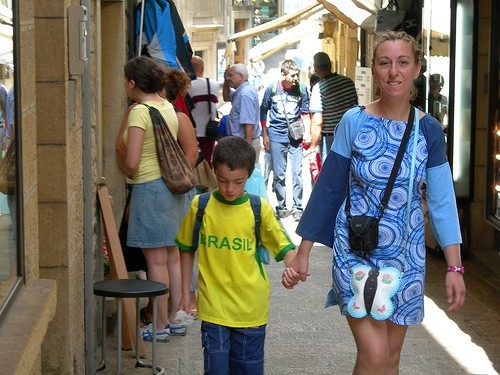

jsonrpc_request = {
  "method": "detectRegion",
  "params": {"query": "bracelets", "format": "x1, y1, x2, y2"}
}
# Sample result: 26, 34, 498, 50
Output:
447, 266, 464, 276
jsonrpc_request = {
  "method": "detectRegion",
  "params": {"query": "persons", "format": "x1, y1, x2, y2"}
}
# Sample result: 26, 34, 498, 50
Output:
306, 52, 359, 167
280, 31, 466, 375
0, 82, 18, 279
124, 55, 187, 344
174, 136, 310, 375
410, 57, 448, 139
118, 56, 320, 326
260, 59, 312, 221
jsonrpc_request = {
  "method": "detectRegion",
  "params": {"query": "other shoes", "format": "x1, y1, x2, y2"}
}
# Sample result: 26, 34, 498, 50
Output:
278, 210, 288, 217
294, 212, 302, 220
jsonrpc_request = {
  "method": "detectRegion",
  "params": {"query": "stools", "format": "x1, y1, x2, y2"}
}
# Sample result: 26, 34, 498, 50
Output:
94, 278, 169, 375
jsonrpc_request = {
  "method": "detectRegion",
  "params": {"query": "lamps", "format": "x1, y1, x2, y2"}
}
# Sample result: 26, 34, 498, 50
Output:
324, 12, 336, 37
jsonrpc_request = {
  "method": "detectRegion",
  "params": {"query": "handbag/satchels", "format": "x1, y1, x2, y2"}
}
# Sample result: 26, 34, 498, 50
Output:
206, 120, 220, 140
349, 214, 378, 252
144, 104, 197, 194
348, 264, 402, 320
289, 120, 305, 140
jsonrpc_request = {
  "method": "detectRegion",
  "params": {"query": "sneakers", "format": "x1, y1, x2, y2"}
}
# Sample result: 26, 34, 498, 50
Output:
168, 322, 186, 335
141, 324, 171, 341
